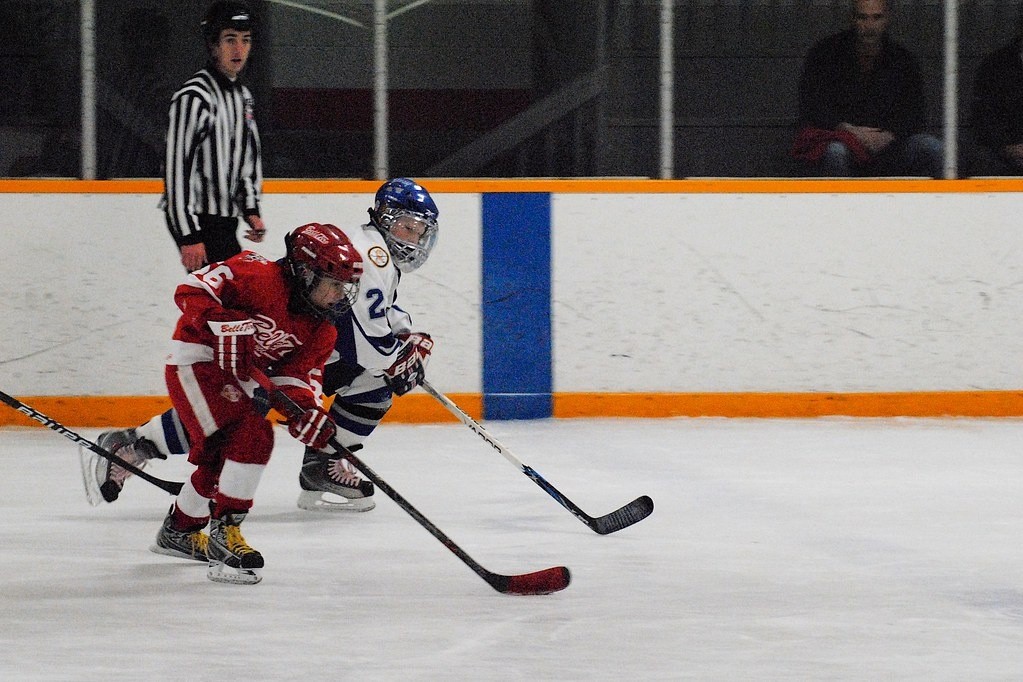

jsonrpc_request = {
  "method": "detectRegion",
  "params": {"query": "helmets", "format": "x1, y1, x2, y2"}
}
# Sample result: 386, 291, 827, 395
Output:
367, 177, 439, 273
281, 223, 364, 325
200, 1, 259, 48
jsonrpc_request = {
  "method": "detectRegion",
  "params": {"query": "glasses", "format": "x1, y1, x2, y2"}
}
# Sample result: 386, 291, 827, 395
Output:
855, 13, 884, 21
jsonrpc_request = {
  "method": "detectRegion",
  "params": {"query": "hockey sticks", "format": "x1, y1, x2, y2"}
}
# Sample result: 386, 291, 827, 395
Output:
419, 378, 654, 535
246, 364, 571, 596
0, 390, 185, 495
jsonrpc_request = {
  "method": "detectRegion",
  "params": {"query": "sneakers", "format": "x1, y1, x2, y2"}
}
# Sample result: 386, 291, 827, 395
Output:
147, 504, 209, 562
79, 427, 167, 508
208, 499, 264, 585
296, 444, 376, 513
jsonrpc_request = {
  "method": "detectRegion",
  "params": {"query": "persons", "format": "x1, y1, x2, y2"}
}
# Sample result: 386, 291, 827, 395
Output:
796, 0, 944, 178
158, 0, 266, 276
968, 40, 1023, 177
79, 178, 440, 516
145, 223, 368, 585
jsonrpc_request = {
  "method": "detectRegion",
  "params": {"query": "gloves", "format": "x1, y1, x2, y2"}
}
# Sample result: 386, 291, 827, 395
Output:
207, 318, 257, 382
374, 332, 434, 397
276, 400, 337, 449
